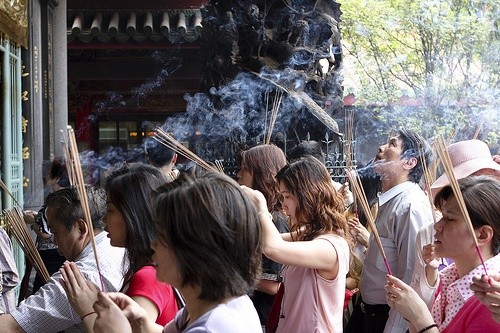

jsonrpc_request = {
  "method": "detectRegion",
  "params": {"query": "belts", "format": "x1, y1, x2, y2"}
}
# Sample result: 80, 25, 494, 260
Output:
359, 296, 389, 314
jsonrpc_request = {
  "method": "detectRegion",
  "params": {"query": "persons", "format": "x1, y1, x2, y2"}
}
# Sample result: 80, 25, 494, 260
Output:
0, 130, 500, 333
240, 155, 366, 333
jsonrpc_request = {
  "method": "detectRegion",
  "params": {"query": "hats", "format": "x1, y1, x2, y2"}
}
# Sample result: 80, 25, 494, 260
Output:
430, 139, 500, 203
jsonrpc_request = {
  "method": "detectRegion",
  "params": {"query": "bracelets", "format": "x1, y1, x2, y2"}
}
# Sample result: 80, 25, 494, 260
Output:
418, 324, 438, 333
257, 209, 273, 219
81, 311, 96, 320
